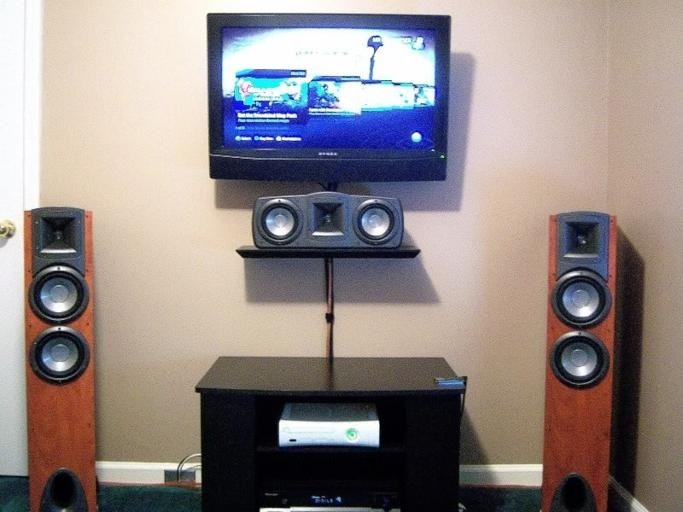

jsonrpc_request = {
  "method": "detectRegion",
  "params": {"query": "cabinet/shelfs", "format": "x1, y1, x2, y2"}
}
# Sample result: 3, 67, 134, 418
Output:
195, 355, 465, 510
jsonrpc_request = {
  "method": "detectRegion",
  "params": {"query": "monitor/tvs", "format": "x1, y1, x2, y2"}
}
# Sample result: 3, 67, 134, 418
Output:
206, 13, 451, 183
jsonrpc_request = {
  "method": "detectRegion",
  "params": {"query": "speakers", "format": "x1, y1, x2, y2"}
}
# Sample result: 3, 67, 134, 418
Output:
252, 191, 405, 250
23, 207, 99, 512
540, 210, 617, 511
